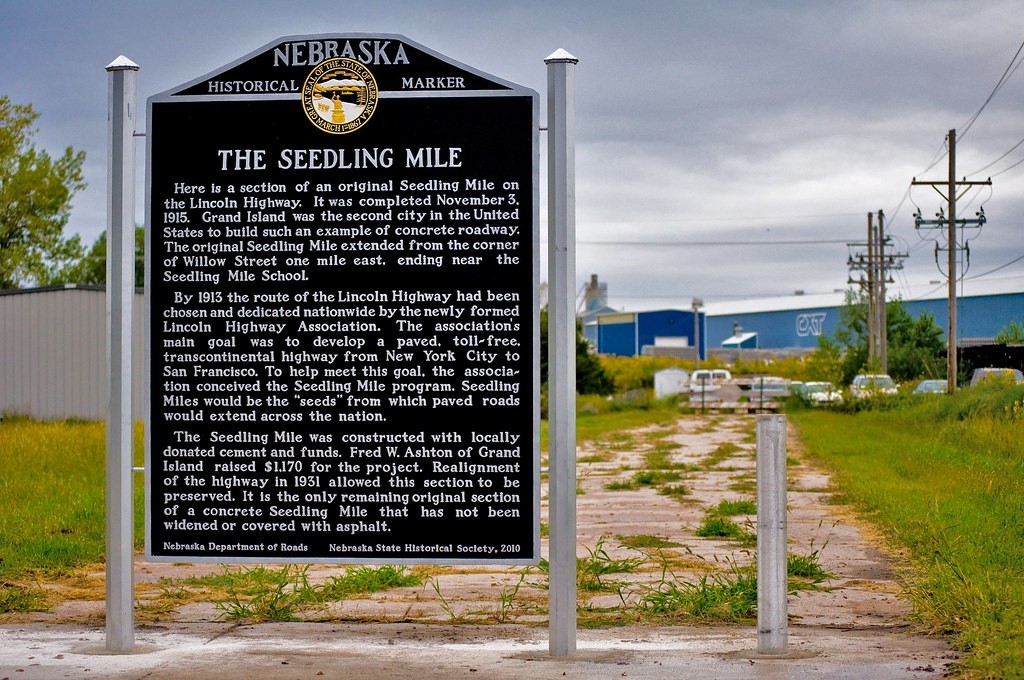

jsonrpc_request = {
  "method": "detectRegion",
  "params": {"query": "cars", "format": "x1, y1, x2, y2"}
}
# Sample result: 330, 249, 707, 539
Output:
851, 373, 902, 400
799, 381, 844, 410
786, 381, 806, 400
748, 377, 786, 413
913, 378, 960, 398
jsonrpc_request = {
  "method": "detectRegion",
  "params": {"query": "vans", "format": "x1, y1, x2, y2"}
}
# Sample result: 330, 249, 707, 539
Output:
683, 368, 736, 410
970, 367, 1023, 390
